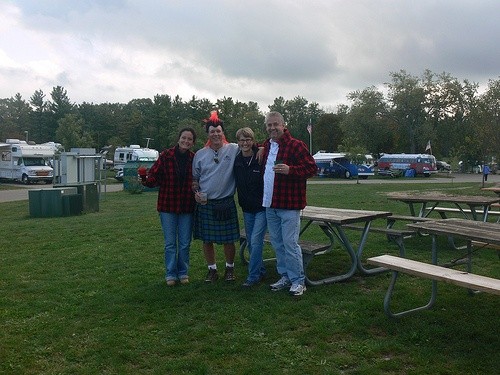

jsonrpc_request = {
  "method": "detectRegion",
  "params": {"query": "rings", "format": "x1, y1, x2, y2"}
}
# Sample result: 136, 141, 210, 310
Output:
280, 169, 281, 170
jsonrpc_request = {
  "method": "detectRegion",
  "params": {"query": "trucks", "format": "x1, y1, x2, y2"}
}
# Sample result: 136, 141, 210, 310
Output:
0, 139, 65, 185
114, 145, 159, 173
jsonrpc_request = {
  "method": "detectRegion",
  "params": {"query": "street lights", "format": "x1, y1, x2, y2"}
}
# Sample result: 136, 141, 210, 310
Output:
24, 131, 28, 143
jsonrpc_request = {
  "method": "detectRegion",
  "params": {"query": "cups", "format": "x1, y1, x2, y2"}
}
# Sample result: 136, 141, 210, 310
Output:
274, 160, 282, 176
200, 193, 207, 206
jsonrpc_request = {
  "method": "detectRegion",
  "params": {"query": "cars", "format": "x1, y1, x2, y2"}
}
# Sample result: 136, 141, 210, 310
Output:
437, 160, 450, 171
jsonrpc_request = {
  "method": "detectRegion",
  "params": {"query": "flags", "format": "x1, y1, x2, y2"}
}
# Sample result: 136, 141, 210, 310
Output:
425, 140, 431, 151
307, 121, 311, 133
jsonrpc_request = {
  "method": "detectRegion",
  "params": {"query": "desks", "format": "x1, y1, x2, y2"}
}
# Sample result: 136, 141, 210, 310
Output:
406, 218, 500, 316
301, 204, 392, 286
388, 190, 500, 250
481, 187, 500, 195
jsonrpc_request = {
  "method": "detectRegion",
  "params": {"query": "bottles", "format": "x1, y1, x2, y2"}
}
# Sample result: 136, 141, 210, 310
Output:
139, 168, 147, 184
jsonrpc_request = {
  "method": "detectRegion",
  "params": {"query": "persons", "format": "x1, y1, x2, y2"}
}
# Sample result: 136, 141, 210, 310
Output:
232, 127, 267, 287
261, 111, 318, 296
483, 164, 489, 181
137, 128, 199, 286
191, 110, 265, 282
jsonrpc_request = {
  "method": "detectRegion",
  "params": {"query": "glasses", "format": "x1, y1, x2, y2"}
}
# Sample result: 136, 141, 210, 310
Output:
238, 138, 252, 142
214, 150, 219, 164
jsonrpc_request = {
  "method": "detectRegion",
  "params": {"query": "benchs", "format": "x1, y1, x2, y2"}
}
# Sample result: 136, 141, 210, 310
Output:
314, 204, 500, 274
367, 254, 500, 317
240, 231, 333, 286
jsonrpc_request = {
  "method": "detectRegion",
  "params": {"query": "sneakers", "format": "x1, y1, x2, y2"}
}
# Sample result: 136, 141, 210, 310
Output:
269, 276, 292, 291
289, 283, 306, 296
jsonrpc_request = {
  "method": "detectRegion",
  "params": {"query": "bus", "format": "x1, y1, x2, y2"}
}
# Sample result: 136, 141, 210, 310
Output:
377, 153, 438, 178
312, 154, 374, 179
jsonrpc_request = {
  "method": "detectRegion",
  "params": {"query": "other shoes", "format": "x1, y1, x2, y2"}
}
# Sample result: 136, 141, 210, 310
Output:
207, 267, 219, 281
167, 280, 176, 286
224, 265, 235, 281
180, 278, 189, 284
242, 280, 256, 287
